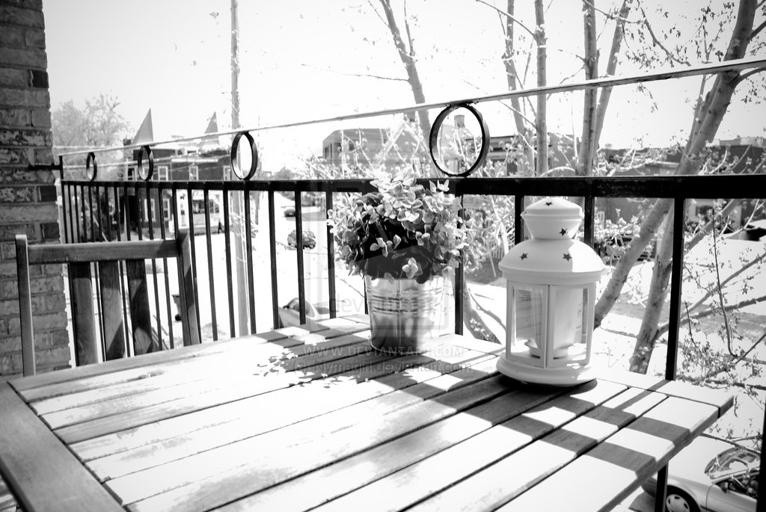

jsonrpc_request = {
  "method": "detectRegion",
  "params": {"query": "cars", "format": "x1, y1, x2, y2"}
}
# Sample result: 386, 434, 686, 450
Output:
640, 434, 762, 511
287, 229, 315, 249
278, 297, 346, 326
285, 208, 296, 217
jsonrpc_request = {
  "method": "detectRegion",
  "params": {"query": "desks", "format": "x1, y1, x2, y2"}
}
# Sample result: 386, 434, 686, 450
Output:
0, 305, 736, 511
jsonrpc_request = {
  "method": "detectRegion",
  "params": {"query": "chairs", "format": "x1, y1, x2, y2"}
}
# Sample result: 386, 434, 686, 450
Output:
12, 227, 204, 379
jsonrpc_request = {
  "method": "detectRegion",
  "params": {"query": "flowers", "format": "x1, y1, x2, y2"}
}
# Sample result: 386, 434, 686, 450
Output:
325, 169, 478, 283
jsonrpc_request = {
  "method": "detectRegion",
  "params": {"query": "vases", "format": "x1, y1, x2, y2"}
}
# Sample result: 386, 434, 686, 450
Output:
362, 270, 443, 358
